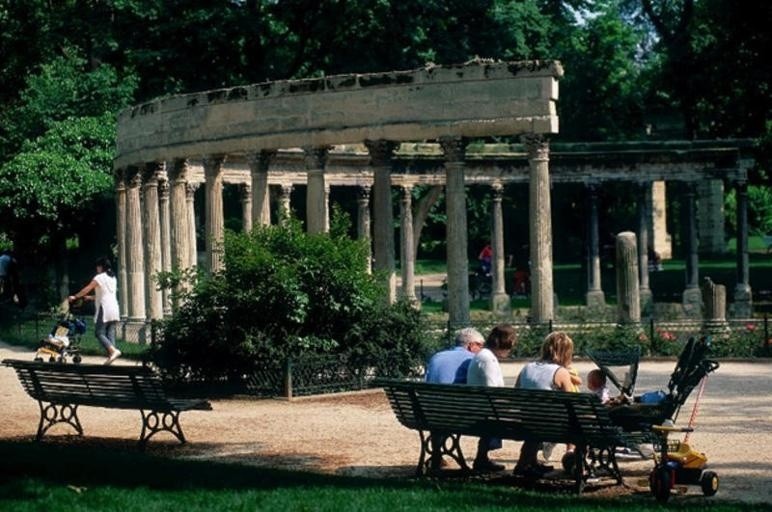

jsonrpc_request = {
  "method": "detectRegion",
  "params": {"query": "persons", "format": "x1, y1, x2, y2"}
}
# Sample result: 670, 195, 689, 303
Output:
508, 240, 530, 295
465, 324, 555, 475
513, 331, 612, 477
478, 242, 492, 273
425, 327, 506, 474
0, 248, 17, 293
69, 256, 122, 366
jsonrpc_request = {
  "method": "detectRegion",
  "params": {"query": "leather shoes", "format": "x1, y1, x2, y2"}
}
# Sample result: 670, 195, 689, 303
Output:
473, 459, 505, 470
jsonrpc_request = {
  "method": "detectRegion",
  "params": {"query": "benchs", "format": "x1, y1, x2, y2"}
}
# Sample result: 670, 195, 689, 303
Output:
0, 359, 213, 452
373, 376, 655, 497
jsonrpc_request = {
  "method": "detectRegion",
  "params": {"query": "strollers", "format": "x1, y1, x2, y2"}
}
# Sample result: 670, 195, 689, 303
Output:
438, 271, 490, 299
34, 298, 87, 364
585, 330, 718, 460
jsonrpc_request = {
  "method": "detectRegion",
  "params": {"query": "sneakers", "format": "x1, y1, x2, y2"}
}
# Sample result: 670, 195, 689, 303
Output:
104, 349, 121, 366
513, 463, 553, 475
